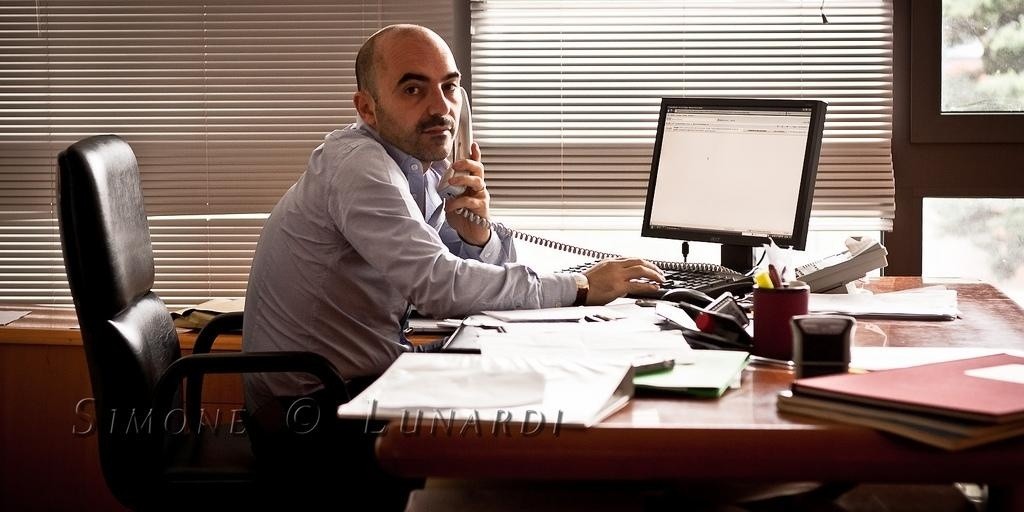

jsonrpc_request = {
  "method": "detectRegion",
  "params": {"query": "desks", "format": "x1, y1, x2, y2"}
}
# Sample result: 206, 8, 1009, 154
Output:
359, 274, 1024, 512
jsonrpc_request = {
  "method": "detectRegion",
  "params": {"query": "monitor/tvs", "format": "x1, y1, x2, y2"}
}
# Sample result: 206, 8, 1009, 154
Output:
641, 98, 828, 276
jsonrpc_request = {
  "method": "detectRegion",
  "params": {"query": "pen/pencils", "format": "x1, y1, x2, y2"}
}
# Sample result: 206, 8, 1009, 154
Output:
755, 264, 786, 289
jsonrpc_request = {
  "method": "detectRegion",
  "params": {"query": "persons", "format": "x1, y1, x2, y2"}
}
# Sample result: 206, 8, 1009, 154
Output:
238, 21, 667, 460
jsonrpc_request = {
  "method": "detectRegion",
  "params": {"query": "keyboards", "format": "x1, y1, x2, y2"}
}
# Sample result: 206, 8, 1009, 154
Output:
554, 260, 753, 300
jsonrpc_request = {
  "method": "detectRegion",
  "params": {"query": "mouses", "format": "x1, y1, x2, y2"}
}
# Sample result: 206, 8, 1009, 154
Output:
661, 288, 715, 308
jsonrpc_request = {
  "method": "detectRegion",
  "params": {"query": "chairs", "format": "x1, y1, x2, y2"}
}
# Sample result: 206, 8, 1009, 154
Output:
50, 131, 432, 512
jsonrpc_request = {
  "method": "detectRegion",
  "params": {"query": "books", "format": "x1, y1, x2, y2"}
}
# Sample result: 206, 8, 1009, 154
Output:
318, 300, 755, 432
775, 350, 1024, 451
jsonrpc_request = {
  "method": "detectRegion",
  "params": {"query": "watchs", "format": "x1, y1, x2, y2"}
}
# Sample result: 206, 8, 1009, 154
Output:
570, 272, 590, 305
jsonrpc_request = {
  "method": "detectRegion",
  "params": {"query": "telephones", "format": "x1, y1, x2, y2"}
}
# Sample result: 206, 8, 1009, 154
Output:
437, 86, 473, 200
